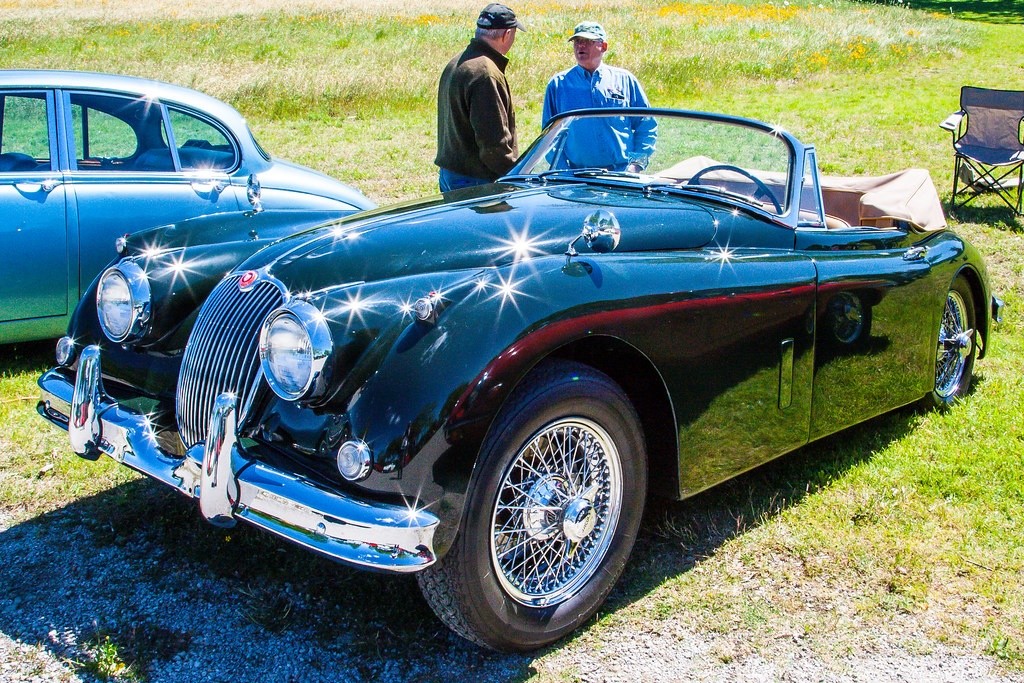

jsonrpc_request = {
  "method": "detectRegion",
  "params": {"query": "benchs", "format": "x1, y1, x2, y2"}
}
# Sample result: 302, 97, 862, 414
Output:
654, 155, 949, 238
131, 145, 235, 176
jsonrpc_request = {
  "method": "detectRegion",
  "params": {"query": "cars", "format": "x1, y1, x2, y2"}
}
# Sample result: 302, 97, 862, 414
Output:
38, 104, 1003, 650
0, 68, 384, 357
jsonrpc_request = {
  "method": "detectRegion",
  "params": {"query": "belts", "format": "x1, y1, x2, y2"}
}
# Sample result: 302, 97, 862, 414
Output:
563, 153, 628, 171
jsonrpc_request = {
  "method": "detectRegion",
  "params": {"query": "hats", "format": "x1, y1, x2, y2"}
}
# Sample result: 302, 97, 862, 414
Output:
568, 22, 606, 42
477, 3, 527, 33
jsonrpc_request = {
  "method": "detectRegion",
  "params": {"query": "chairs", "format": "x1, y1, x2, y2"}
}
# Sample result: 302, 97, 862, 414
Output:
940, 85, 1024, 219
0, 152, 39, 172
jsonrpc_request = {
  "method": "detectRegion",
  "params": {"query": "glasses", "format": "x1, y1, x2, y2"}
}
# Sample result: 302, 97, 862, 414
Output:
571, 37, 602, 44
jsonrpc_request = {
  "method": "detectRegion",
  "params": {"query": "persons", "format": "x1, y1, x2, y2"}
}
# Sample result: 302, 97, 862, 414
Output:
434, 3, 527, 193
468, 201, 519, 214
542, 22, 658, 174
